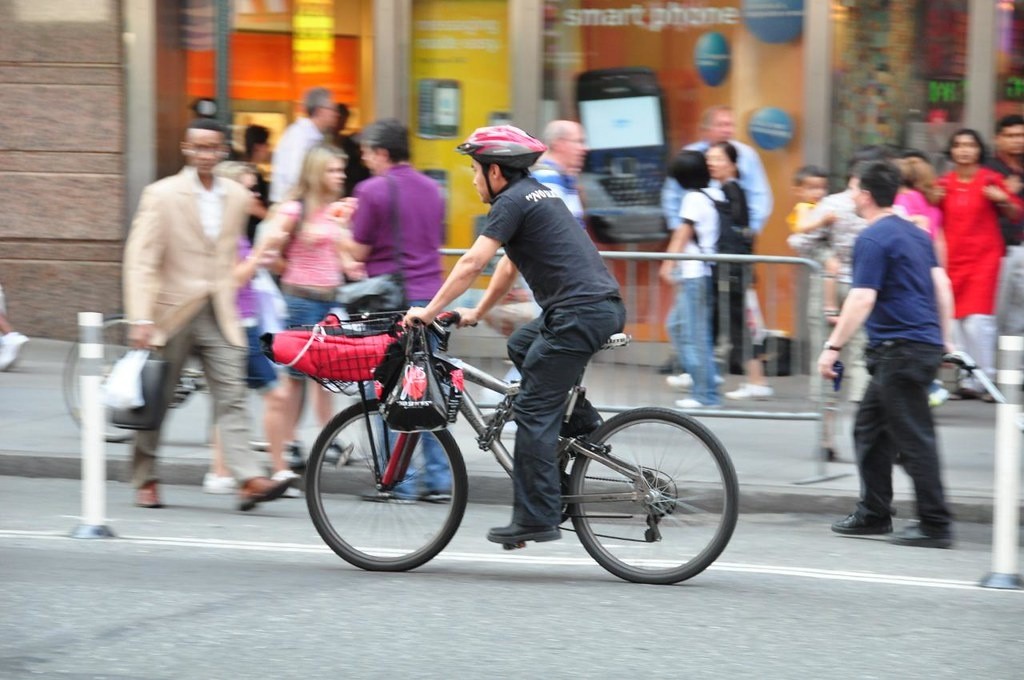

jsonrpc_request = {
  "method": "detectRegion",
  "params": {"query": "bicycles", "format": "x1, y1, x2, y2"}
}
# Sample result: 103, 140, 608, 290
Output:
292, 304, 743, 586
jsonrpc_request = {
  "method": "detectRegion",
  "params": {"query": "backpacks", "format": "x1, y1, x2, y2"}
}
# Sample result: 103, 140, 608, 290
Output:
686, 188, 751, 283
259, 313, 405, 383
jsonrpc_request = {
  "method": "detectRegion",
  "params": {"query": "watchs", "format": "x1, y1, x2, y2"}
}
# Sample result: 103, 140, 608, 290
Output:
823, 342, 841, 352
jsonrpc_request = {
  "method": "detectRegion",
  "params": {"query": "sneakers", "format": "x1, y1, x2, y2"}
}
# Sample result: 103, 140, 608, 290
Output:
272, 470, 300, 496
417, 491, 454, 503
323, 442, 351, 466
726, 383, 775, 399
667, 374, 695, 389
285, 442, 305, 472
204, 473, 238, 495
360, 488, 415, 505
678, 397, 718, 409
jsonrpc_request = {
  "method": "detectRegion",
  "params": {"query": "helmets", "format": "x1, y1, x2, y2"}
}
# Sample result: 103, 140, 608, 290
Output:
456, 125, 546, 170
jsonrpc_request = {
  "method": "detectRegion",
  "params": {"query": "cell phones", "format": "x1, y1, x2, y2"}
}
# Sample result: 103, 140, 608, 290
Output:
413, 78, 461, 141
420, 168, 448, 198
574, 67, 674, 241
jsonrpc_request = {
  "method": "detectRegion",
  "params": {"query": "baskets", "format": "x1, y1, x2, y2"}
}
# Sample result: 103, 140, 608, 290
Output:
287, 316, 401, 397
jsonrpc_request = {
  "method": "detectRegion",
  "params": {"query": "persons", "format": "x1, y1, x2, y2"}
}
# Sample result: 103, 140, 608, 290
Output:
402, 125, 626, 545
202, 161, 302, 493
260, 142, 362, 465
335, 117, 454, 504
659, 104, 775, 410
786, 113, 1024, 460
484, 120, 588, 437
830, 160, 955, 550
121, 118, 288, 511
187, 89, 377, 250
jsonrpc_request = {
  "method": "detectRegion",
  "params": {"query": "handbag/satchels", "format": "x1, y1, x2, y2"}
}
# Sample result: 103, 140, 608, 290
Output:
111, 346, 170, 431
334, 274, 405, 319
372, 323, 463, 433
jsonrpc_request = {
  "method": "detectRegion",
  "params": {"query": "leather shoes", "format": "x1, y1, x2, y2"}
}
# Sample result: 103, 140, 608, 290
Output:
834, 514, 892, 535
487, 521, 562, 544
237, 476, 292, 511
134, 482, 162, 508
572, 417, 604, 435
893, 526, 948, 547
